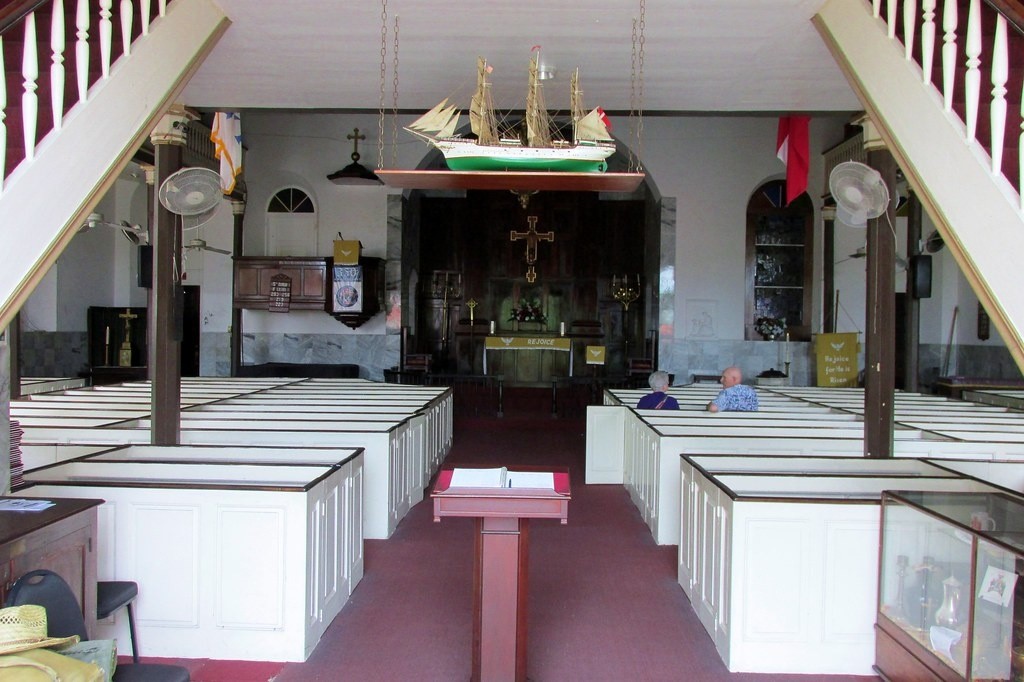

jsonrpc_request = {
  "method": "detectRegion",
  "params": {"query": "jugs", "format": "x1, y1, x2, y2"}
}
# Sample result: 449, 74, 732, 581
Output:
934, 572, 965, 631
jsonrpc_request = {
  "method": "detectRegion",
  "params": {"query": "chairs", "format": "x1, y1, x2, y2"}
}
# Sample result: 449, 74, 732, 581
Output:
402, 326, 432, 372
627, 329, 656, 375
6, 570, 190, 682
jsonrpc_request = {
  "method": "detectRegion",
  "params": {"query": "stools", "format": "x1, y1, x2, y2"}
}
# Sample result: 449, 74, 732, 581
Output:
96, 580, 138, 663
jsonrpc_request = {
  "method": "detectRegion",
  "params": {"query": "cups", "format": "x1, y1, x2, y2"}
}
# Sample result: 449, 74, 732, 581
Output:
970, 512, 997, 535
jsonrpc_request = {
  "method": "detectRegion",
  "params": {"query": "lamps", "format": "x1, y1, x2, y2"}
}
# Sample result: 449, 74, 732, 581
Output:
787, 332, 789, 362
327, 128, 385, 186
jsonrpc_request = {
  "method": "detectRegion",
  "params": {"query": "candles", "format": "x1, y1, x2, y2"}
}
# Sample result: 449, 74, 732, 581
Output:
491, 321, 495, 336
612, 273, 641, 287
561, 321, 565, 337
432, 270, 462, 284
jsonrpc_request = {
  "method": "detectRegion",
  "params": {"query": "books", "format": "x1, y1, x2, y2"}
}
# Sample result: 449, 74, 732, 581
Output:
10, 420, 26, 488
449, 466, 555, 490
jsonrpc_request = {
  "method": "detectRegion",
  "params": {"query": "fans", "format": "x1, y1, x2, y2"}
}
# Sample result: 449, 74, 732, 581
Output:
77, 210, 139, 235
828, 161, 889, 220
157, 167, 231, 256
836, 204, 867, 227
120, 220, 149, 244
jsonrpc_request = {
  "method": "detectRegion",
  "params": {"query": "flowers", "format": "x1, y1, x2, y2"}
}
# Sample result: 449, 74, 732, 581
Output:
508, 298, 546, 322
754, 317, 785, 337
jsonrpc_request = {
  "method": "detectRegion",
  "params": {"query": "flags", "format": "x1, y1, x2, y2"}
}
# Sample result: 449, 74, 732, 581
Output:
776, 115, 811, 207
209, 112, 243, 196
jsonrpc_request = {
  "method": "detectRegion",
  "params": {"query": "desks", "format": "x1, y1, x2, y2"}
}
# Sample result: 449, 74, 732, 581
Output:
484, 334, 574, 393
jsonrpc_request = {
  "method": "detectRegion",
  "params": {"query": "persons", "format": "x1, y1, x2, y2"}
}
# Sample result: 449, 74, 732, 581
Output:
637, 370, 680, 411
707, 367, 758, 412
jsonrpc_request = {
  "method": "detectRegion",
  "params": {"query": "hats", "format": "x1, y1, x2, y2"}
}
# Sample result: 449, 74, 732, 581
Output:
0, 605, 80, 655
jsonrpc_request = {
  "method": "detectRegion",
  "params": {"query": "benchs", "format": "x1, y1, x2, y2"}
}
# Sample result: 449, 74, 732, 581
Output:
8, 376, 452, 666
602, 385, 1024, 675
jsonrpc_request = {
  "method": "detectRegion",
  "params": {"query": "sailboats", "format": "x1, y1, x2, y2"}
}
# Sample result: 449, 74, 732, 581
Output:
398, 44, 618, 174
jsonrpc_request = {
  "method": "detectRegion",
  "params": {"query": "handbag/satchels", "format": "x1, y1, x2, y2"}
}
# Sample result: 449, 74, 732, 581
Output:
0, 649, 106, 682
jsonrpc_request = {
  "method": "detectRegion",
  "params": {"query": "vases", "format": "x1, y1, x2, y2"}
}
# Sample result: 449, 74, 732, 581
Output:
764, 333, 776, 341
519, 321, 539, 331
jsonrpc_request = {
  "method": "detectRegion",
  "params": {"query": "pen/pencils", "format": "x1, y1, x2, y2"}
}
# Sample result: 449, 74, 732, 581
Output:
509, 479, 511, 488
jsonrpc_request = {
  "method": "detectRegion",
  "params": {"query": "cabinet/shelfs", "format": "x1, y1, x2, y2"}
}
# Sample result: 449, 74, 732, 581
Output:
232, 256, 385, 313
